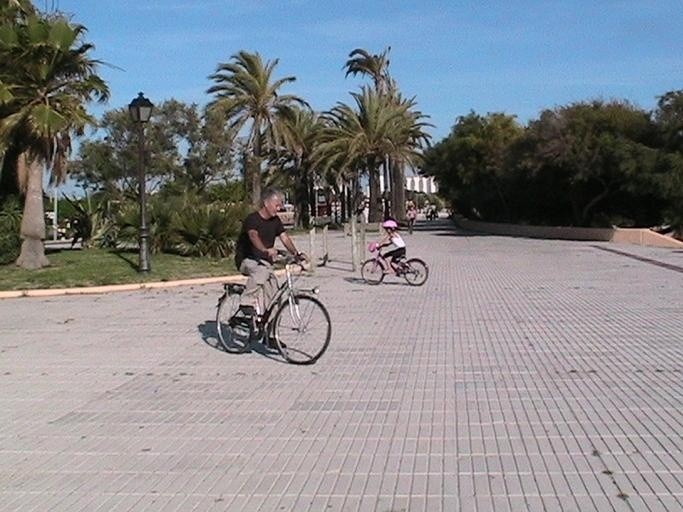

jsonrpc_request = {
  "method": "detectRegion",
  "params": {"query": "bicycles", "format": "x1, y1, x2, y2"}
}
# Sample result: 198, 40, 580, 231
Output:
360, 243, 428, 286
212, 250, 333, 365
407, 217, 415, 234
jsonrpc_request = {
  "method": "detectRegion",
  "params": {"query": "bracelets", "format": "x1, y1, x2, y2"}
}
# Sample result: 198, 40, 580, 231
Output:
290, 249, 295, 252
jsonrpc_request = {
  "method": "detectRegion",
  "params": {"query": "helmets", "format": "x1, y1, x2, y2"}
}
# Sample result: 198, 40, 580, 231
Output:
382, 220, 397, 228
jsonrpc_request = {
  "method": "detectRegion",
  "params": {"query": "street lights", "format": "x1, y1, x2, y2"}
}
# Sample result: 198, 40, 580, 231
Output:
128, 90, 154, 274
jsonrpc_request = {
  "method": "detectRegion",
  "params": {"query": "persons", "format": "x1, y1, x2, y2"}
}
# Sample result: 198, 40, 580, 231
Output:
378, 220, 405, 274
405, 200, 416, 234
234, 187, 311, 348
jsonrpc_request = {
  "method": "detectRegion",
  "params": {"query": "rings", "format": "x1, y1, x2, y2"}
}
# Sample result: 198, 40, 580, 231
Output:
303, 256, 305, 259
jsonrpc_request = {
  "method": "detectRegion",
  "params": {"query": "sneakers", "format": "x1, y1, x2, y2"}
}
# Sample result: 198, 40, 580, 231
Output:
240, 304, 257, 316
262, 336, 286, 349
